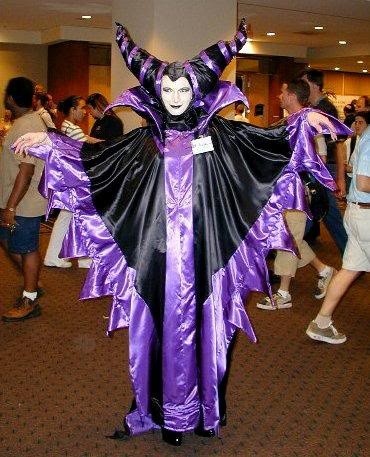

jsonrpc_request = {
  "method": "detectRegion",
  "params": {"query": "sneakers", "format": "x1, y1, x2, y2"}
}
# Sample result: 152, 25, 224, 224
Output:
306, 320, 348, 344
315, 264, 338, 299
256, 294, 293, 310
1, 296, 42, 321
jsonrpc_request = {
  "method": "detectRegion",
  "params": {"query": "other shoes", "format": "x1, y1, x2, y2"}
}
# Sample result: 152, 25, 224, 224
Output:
43, 257, 73, 268
160, 427, 182, 446
197, 423, 216, 438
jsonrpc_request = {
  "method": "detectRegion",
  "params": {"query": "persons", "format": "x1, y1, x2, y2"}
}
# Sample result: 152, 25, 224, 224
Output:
256, 69, 370, 344
11, 61, 338, 445
0, 75, 124, 323
235, 105, 249, 123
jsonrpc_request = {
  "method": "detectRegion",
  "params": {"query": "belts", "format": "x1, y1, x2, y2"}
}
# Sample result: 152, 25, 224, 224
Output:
351, 202, 370, 209
